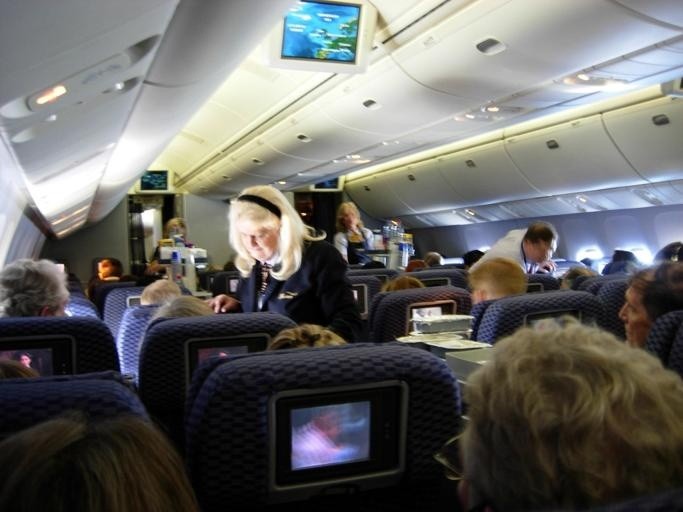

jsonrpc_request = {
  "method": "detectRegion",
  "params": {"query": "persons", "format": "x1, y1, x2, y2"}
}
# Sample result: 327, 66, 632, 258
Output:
151, 217, 194, 277
0, 242, 682, 511
468, 221, 559, 274
333, 201, 386, 264
205, 185, 368, 343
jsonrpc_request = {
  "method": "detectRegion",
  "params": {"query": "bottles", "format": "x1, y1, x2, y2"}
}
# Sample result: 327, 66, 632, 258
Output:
170, 250, 196, 291
383, 226, 415, 267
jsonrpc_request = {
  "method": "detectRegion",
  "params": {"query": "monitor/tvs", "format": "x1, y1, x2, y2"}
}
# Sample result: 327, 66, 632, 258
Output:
310, 175, 345, 191
0, 335, 73, 380
525, 309, 583, 337
275, 0, 378, 73
406, 300, 456, 336
136, 169, 172, 193
185, 332, 272, 394
267, 381, 409, 490
